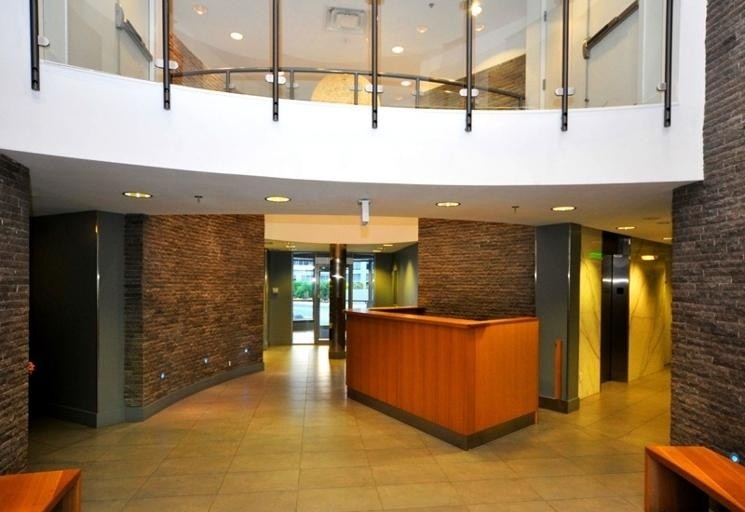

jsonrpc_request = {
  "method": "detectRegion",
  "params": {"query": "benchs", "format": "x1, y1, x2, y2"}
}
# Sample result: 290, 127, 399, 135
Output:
643, 445, 745, 512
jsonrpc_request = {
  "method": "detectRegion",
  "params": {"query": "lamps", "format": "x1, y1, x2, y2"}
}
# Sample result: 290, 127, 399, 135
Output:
324, 7, 366, 39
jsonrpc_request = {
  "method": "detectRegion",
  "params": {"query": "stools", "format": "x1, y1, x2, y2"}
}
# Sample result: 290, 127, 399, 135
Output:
0, 468, 81, 512
